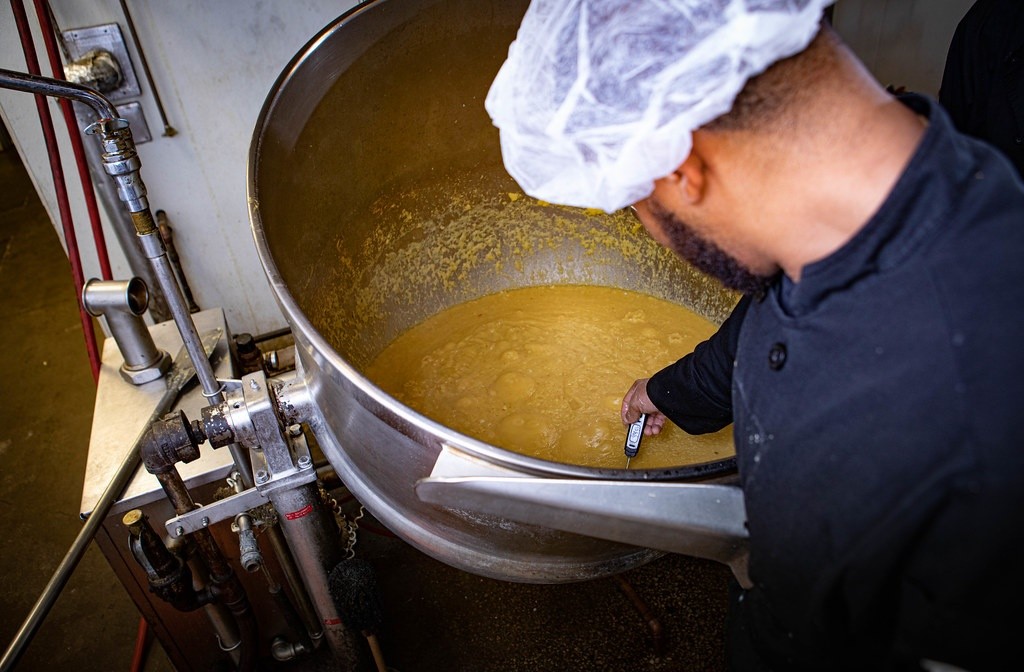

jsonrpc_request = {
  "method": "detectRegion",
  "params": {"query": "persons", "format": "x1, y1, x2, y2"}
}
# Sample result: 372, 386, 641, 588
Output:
483, 0, 1024, 672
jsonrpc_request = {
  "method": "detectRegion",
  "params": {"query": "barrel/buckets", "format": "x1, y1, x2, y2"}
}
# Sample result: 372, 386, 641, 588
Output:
244, 0, 740, 582
